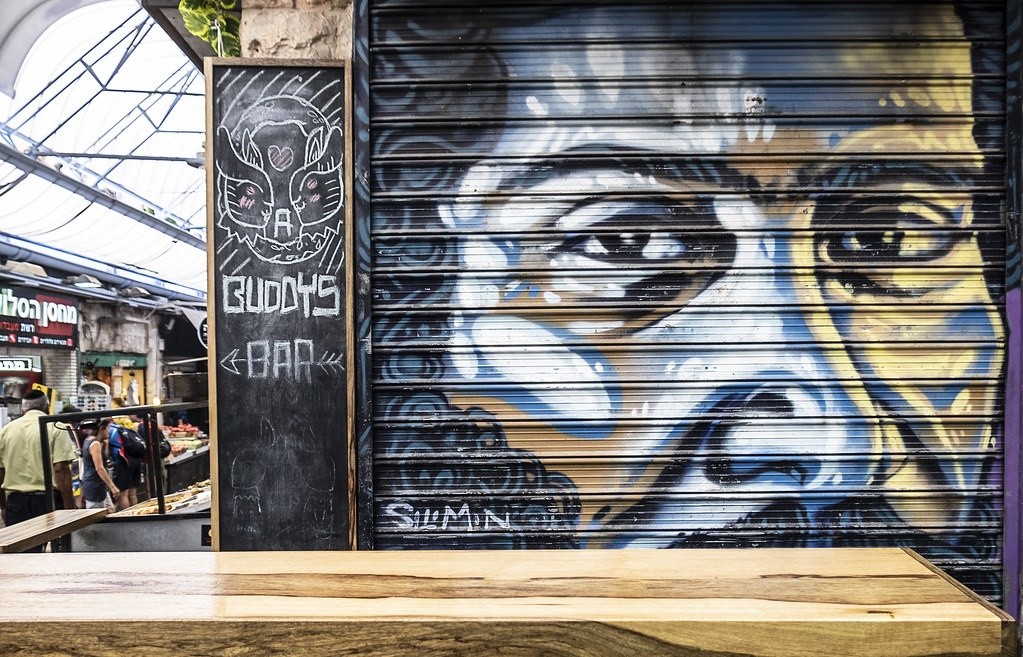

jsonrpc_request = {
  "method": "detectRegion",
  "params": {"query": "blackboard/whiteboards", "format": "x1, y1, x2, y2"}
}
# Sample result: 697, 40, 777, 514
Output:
201, 54, 358, 551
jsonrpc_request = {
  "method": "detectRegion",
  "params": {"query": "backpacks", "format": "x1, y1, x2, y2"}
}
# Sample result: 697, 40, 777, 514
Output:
108, 425, 147, 458
140, 420, 171, 460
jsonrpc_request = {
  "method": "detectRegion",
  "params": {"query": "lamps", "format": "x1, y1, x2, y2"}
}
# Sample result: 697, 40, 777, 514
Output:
119, 287, 151, 298
4, 257, 50, 279
63, 273, 103, 288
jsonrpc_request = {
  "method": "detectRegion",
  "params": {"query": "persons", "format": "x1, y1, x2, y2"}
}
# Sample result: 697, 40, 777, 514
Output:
0, 390, 76, 554
55, 401, 170, 514
